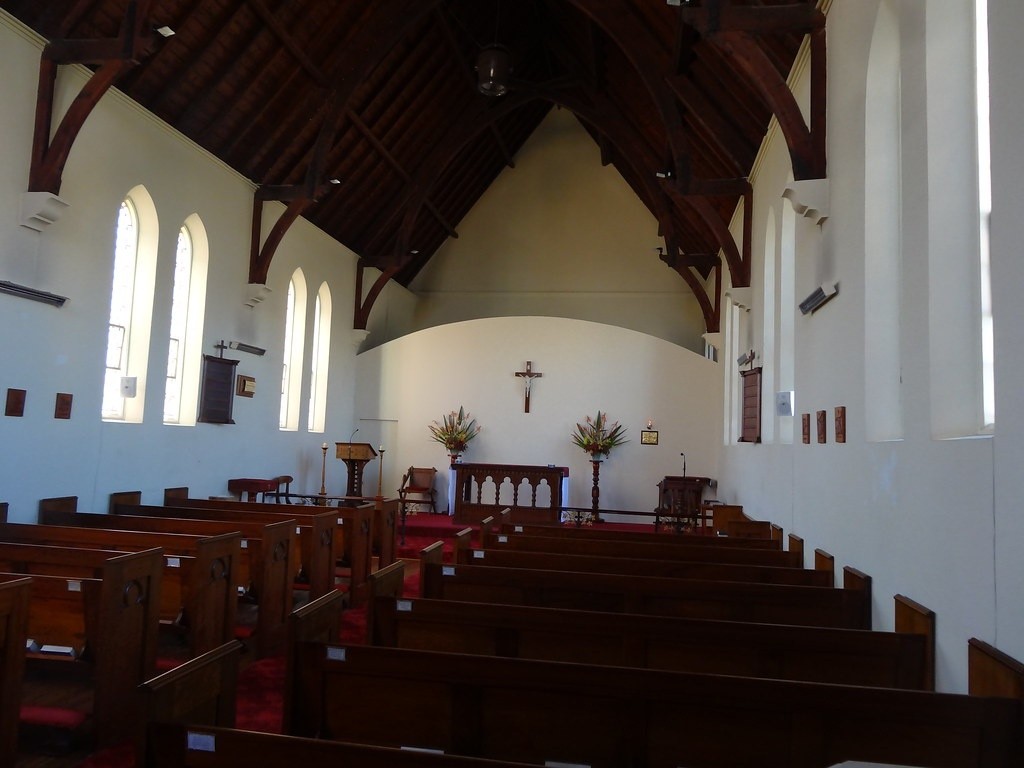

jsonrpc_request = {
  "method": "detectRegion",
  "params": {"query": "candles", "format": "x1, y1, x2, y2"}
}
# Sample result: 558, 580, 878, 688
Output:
380, 446, 383, 450
323, 443, 327, 447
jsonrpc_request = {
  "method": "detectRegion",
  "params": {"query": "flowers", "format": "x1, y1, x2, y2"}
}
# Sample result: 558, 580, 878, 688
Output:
562, 510, 596, 528
571, 411, 630, 459
430, 407, 481, 452
659, 515, 697, 535
400, 502, 420, 516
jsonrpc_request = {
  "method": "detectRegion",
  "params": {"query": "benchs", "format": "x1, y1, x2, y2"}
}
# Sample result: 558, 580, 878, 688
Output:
0, 487, 399, 768
137, 508, 1024, 768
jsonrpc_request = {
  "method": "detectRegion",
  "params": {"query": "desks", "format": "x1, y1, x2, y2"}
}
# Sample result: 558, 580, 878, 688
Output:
451, 463, 569, 527
228, 479, 277, 503
657, 476, 707, 516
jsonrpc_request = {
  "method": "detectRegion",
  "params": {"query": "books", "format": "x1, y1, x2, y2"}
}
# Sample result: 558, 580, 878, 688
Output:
27, 639, 78, 658
717, 530, 728, 537
237, 586, 246, 597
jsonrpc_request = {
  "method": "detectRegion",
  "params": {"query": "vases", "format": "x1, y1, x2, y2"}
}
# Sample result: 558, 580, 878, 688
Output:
450, 449, 458, 454
592, 451, 601, 460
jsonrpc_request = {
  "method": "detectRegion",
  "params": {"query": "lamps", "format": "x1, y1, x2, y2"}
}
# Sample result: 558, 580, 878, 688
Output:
0, 280, 71, 309
799, 282, 837, 315
229, 341, 266, 355
737, 352, 748, 366
473, 0, 517, 97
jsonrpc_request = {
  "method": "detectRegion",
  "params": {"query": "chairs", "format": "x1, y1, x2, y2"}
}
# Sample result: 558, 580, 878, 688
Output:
274, 476, 293, 504
397, 466, 437, 515
